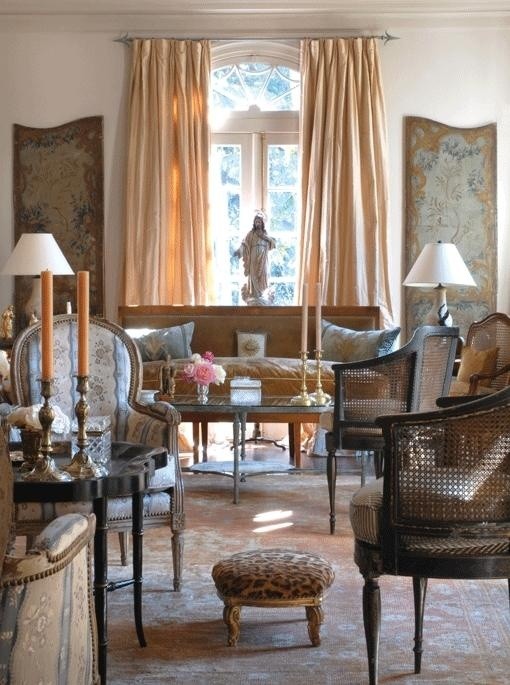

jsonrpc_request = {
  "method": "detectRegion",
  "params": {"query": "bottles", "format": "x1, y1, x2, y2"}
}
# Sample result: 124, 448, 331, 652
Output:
160, 355, 174, 401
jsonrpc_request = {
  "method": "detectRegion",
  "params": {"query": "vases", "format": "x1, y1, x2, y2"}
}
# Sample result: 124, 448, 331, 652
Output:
19, 431, 47, 470
196, 383, 210, 402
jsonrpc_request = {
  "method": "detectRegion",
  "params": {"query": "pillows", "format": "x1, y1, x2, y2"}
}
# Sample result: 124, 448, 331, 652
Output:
235, 331, 268, 357
320, 317, 401, 366
135, 321, 195, 362
455, 347, 501, 384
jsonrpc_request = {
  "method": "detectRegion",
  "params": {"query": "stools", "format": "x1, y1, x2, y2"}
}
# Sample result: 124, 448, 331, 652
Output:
212, 548, 336, 649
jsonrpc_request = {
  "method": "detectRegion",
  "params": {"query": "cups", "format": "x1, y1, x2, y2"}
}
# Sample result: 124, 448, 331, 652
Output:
20, 428, 40, 465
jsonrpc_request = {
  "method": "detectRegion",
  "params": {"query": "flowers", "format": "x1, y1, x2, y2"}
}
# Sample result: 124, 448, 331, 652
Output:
5, 403, 70, 434
182, 350, 226, 386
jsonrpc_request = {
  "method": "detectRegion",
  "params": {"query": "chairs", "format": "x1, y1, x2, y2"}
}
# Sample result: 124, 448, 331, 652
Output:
326, 328, 458, 535
350, 389, 509, 684
449, 313, 510, 404
10, 315, 187, 594
1, 427, 102, 685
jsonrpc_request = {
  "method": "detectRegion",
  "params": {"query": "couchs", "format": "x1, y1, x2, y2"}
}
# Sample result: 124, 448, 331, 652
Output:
119, 305, 382, 470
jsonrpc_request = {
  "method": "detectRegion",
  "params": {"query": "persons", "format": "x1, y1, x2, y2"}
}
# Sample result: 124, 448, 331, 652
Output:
233, 212, 277, 306
2, 304, 15, 339
154, 352, 176, 403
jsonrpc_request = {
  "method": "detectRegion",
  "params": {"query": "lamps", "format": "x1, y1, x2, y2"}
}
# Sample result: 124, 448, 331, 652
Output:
402, 243, 478, 328
2, 233, 76, 321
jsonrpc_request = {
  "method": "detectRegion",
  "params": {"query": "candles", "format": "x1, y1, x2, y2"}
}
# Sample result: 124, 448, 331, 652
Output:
301, 282, 309, 351
315, 282, 322, 351
77, 271, 89, 376
40, 269, 55, 381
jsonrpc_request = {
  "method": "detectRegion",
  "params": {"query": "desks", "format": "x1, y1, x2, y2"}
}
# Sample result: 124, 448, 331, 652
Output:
9, 440, 168, 681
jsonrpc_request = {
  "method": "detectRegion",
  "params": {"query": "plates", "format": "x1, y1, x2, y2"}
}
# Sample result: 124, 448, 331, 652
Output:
8, 451, 50, 463
139, 390, 159, 402
